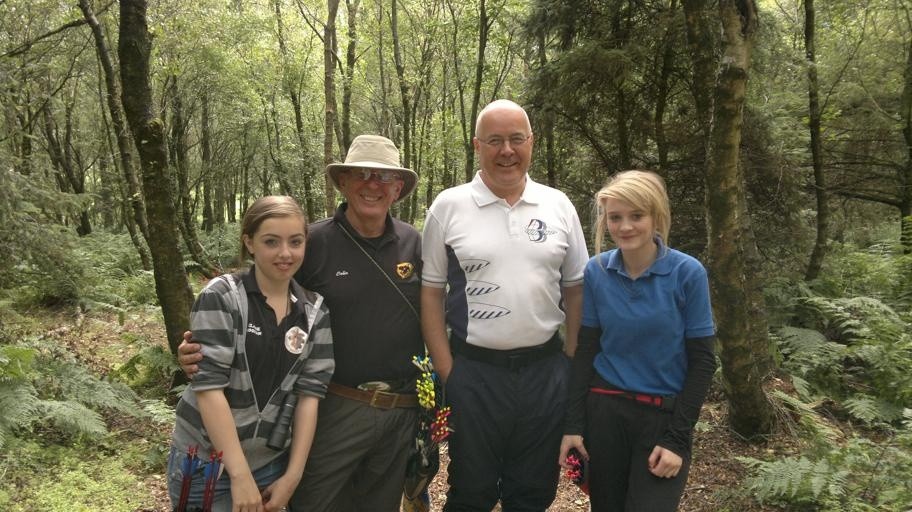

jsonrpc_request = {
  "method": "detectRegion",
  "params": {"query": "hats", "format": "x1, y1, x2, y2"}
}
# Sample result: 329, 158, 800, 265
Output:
327, 135, 418, 204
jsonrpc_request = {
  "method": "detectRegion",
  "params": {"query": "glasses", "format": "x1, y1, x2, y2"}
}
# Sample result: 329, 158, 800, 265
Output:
346, 168, 399, 182
474, 135, 528, 147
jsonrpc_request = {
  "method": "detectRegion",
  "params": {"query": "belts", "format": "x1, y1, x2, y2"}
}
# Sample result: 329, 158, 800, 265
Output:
459, 330, 563, 373
326, 382, 419, 410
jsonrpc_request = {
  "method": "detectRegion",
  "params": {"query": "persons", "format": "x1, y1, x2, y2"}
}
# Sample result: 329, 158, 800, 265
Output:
170, 194, 335, 510
176, 135, 431, 512
418, 100, 588, 510
556, 171, 716, 511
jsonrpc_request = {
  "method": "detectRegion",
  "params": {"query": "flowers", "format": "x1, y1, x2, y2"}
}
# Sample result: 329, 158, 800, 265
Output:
562, 447, 598, 496
418, 402, 450, 457
414, 348, 439, 409
